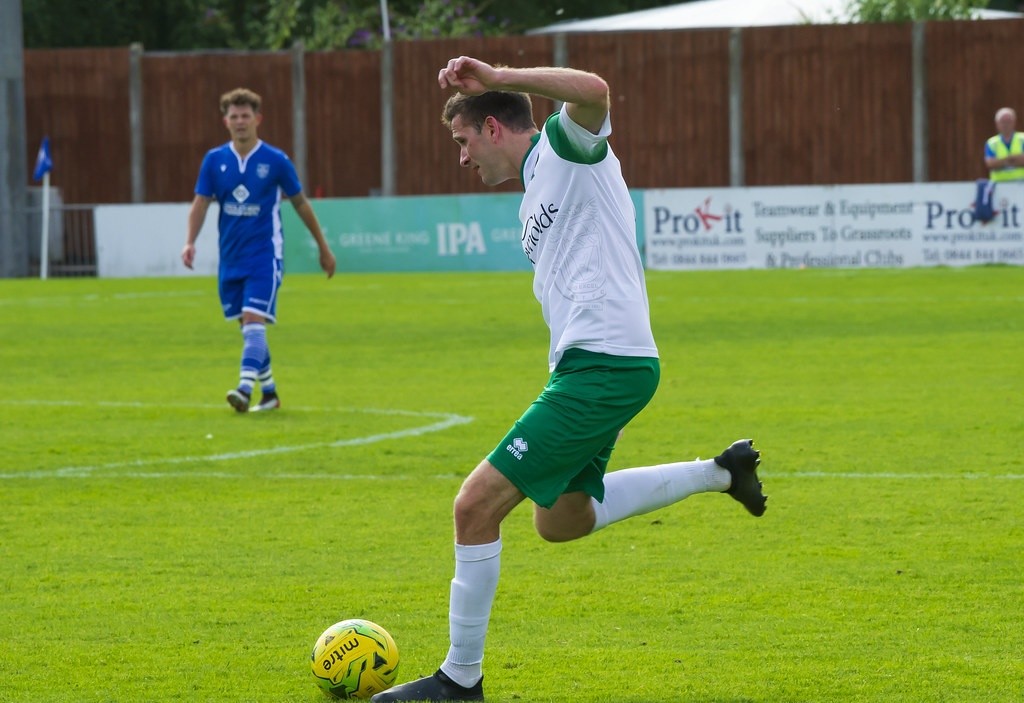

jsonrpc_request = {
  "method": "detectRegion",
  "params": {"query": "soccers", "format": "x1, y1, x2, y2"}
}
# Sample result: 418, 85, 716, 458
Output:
310, 618, 400, 700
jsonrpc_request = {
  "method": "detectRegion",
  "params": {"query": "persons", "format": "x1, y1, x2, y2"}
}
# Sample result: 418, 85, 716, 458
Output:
372, 54, 771, 703
182, 87, 336, 413
984, 107, 1024, 183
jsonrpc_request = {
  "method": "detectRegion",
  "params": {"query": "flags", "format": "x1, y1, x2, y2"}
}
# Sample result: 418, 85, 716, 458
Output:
34, 138, 53, 181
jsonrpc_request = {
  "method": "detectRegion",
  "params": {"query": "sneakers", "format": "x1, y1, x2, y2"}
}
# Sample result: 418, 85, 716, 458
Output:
251, 392, 280, 412
371, 667, 485, 703
715, 438, 767, 517
226, 388, 251, 412
